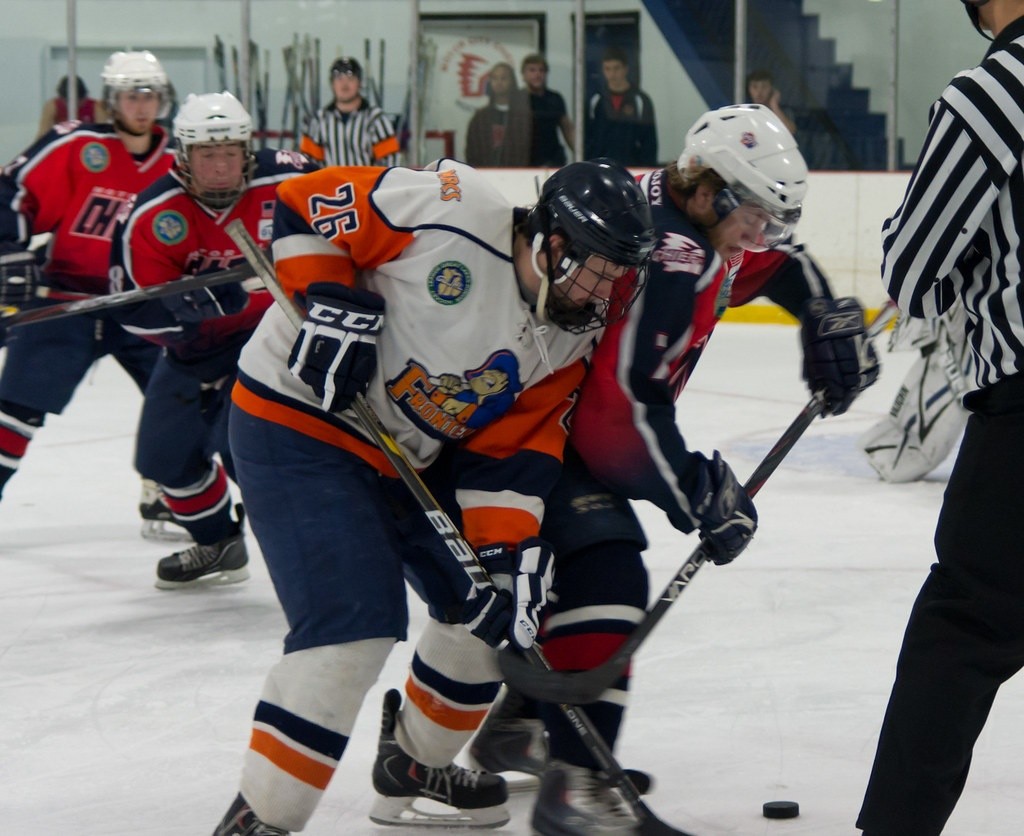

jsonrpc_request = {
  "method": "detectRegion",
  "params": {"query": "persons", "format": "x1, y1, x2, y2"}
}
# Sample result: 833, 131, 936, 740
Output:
37, 73, 104, 148
0, 50, 197, 543
472, 105, 881, 835
584, 55, 658, 169
109, 92, 333, 589
209, 156, 656, 836
465, 62, 532, 169
747, 69, 795, 135
303, 56, 397, 172
518, 53, 574, 170
853, 0, 1024, 836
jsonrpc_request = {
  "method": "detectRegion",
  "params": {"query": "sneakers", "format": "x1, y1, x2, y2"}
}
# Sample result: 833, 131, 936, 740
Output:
153, 504, 250, 590
139, 479, 194, 541
529, 758, 682, 836
367, 687, 511, 828
468, 698, 547, 798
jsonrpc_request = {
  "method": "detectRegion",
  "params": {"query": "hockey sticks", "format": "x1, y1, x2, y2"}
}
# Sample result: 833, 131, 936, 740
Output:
0, 248, 274, 331
214, 32, 429, 169
224, 217, 690, 836
495, 298, 899, 702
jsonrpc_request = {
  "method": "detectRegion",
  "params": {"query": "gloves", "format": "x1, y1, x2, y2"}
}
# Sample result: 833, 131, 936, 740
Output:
799, 293, 883, 418
163, 271, 251, 323
689, 449, 759, 568
287, 279, 386, 414
0, 256, 51, 306
477, 535, 551, 647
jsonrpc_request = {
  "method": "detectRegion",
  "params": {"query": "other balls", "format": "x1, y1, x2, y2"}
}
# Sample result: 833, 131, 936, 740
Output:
763, 801, 799, 819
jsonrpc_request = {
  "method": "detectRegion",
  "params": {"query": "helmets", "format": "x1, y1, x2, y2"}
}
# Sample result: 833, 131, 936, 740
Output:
171, 90, 254, 204
330, 56, 365, 103
529, 154, 659, 335
100, 50, 171, 134
677, 103, 810, 254
